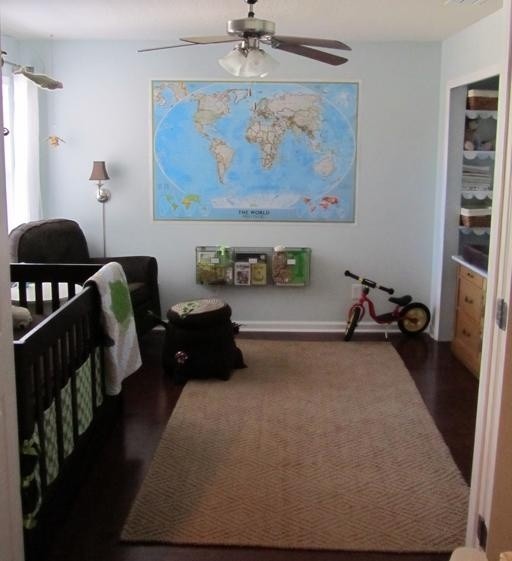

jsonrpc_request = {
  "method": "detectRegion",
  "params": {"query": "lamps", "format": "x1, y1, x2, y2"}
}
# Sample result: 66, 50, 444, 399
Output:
89, 161, 112, 258
218, 47, 281, 81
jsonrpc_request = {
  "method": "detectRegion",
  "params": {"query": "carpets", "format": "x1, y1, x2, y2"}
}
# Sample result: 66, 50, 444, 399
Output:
119, 340, 471, 554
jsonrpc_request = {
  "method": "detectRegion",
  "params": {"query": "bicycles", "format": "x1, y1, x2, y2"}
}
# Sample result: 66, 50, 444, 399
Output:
341, 268, 431, 346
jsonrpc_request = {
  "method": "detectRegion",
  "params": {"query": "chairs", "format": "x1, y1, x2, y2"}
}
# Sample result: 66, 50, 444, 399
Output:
8, 218, 161, 360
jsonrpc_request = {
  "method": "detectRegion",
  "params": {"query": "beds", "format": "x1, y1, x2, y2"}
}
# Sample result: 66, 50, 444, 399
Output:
8, 262, 133, 532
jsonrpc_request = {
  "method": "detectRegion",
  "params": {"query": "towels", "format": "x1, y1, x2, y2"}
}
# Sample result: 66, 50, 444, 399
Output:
82, 262, 142, 396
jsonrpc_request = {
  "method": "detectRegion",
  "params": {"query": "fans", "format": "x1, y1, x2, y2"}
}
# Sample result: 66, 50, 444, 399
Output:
135, 0, 351, 68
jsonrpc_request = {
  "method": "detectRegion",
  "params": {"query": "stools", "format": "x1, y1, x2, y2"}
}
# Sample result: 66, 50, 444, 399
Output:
146, 298, 249, 386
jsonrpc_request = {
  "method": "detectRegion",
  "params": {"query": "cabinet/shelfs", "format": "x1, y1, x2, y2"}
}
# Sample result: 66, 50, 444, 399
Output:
451, 89, 499, 381
